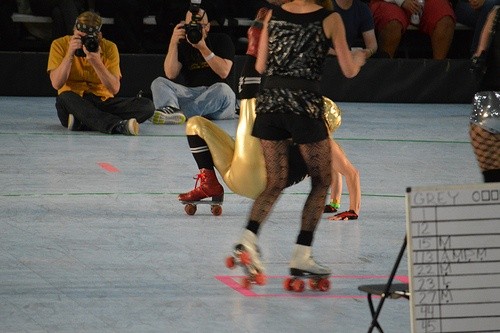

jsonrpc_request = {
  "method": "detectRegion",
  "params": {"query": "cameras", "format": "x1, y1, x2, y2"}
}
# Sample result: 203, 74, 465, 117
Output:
182, 0, 202, 44
75, 35, 98, 57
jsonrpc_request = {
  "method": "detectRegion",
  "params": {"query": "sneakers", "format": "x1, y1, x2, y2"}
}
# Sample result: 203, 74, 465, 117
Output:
68, 114, 80, 131
115, 118, 139, 136
150, 105, 186, 125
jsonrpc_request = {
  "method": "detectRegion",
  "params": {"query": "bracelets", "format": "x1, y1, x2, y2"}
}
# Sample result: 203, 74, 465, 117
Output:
329, 198, 340, 209
204, 52, 215, 61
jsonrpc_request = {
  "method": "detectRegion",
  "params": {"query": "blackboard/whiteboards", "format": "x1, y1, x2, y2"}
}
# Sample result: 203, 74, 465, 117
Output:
404, 182, 499, 333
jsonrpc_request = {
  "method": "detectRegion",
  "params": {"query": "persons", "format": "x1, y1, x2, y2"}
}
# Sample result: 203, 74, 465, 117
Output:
323, 0, 500, 84
47, 12, 155, 136
148, 7, 237, 125
178, 7, 361, 221
227, 0, 368, 292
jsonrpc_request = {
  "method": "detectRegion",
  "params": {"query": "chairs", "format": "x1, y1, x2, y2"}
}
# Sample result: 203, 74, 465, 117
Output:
358, 232, 410, 333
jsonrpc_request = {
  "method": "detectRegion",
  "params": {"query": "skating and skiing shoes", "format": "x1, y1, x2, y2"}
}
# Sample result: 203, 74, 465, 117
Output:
245, 7, 267, 55
178, 168, 225, 216
226, 228, 266, 289
285, 244, 332, 292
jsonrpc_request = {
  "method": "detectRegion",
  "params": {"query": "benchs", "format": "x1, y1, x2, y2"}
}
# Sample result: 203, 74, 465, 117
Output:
11, 14, 472, 30
